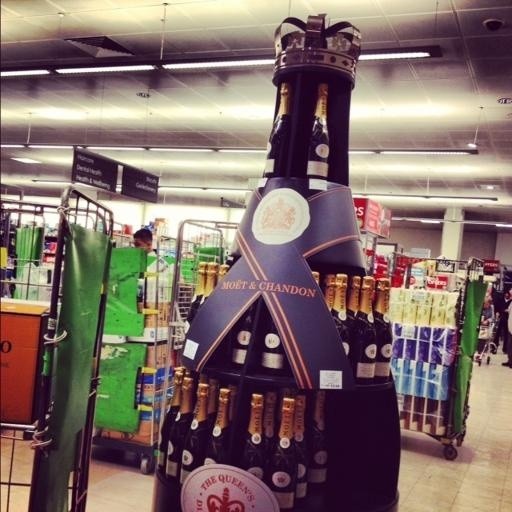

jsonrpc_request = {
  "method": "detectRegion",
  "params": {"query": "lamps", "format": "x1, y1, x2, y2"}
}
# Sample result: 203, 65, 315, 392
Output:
0, 0, 512, 228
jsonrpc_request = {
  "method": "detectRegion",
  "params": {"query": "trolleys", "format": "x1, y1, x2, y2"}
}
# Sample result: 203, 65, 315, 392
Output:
470, 309, 503, 368
86, 232, 198, 475
383, 248, 487, 460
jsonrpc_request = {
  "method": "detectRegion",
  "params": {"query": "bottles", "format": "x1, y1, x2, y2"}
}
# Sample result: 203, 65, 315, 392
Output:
156, 261, 393, 511
264, 82, 330, 179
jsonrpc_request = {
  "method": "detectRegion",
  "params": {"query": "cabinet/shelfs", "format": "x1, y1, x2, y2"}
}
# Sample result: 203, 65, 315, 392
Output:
0, 182, 240, 512
361, 230, 512, 461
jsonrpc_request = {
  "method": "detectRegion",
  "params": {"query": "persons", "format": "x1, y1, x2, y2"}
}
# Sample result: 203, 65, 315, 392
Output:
475, 288, 511, 368
133, 229, 168, 272
